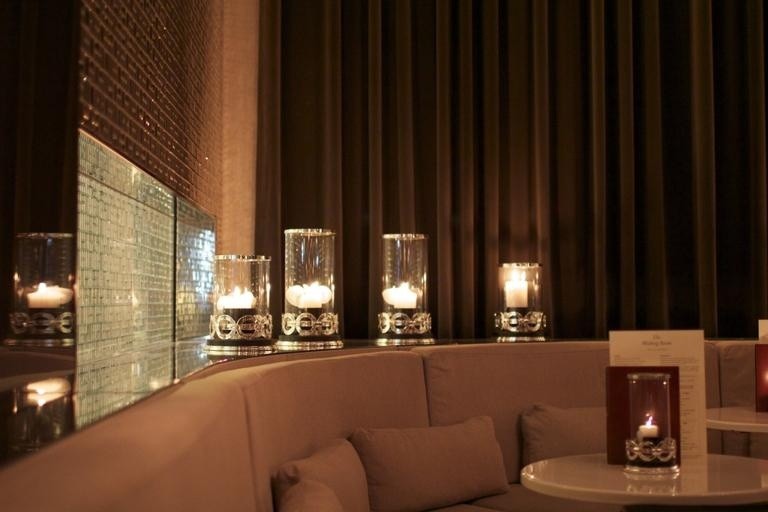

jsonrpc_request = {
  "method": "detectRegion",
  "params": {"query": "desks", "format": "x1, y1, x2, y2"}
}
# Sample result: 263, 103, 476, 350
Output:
518, 405, 767, 511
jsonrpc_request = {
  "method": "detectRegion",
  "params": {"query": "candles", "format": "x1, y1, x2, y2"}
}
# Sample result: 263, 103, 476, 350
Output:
637, 415, 659, 438
26, 376, 72, 406
27, 279, 73, 309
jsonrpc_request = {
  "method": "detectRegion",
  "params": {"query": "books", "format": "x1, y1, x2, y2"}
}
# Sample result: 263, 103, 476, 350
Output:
604, 327, 709, 462
754, 318, 768, 415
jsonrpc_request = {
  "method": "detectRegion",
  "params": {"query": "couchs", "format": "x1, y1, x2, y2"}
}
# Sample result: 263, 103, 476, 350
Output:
0, 337, 768, 512
